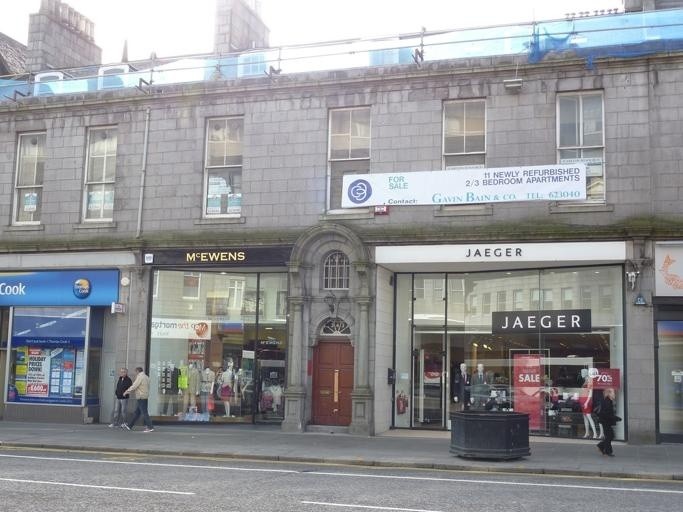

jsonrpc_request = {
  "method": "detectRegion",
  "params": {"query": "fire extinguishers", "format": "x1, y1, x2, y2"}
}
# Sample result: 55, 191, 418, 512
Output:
397, 392, 406, 414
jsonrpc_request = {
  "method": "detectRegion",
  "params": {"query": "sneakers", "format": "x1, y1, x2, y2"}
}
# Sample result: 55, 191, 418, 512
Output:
594, 444, 615, 457
142, 427, 154, 433
119, 423, 131, 431
108, 424, 114, 428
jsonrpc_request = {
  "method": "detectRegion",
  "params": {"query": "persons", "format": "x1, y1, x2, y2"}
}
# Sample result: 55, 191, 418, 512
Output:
454, 363, 491, 412
160, 358, 238, 418
108, 367, 155, 433
597, 387, 624, 457
577, 368, 598, 438
